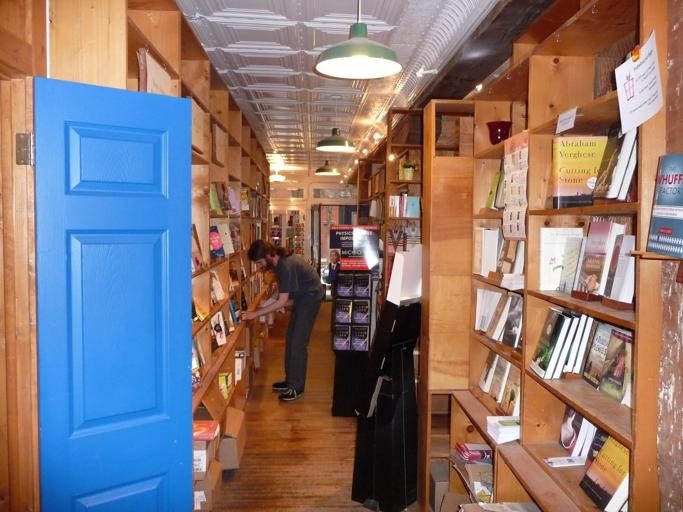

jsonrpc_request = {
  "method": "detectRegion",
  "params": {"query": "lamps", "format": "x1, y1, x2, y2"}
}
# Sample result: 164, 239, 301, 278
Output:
315, 159, 341, 176
316, 95, 357, 152
314, 0, 402, 81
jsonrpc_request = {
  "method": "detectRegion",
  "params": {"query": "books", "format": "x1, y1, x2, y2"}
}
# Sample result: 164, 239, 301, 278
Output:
529, 124, 639, 511
646, 153, 683, 257
334, 274, 372, 351
387, 192, 422, 221
448, 173, 539, 512
192, 182, 270, 399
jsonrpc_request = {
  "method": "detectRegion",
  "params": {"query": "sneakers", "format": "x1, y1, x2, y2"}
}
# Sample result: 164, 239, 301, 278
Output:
272, 381, 289, 390
278, 388, 304, 401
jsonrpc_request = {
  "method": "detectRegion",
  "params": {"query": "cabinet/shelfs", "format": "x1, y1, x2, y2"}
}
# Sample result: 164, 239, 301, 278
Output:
467, 0, 683, 511
442, 390, 585, 511
127, 0, 270, 512
270, 208, 304, 260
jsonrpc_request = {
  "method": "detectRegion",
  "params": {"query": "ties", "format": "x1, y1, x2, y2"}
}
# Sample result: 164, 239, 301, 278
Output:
333, 265, 334, 271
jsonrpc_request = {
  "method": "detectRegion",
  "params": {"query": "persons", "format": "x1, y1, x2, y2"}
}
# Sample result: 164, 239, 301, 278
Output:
241, 239, 325, 401
329, 249, 340, 282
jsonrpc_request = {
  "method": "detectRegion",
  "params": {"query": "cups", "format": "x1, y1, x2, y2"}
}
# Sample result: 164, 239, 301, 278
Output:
486, 121, 513, 144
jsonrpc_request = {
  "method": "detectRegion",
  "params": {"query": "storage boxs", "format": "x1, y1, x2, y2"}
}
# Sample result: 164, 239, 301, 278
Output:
192, 342, 248, 512
